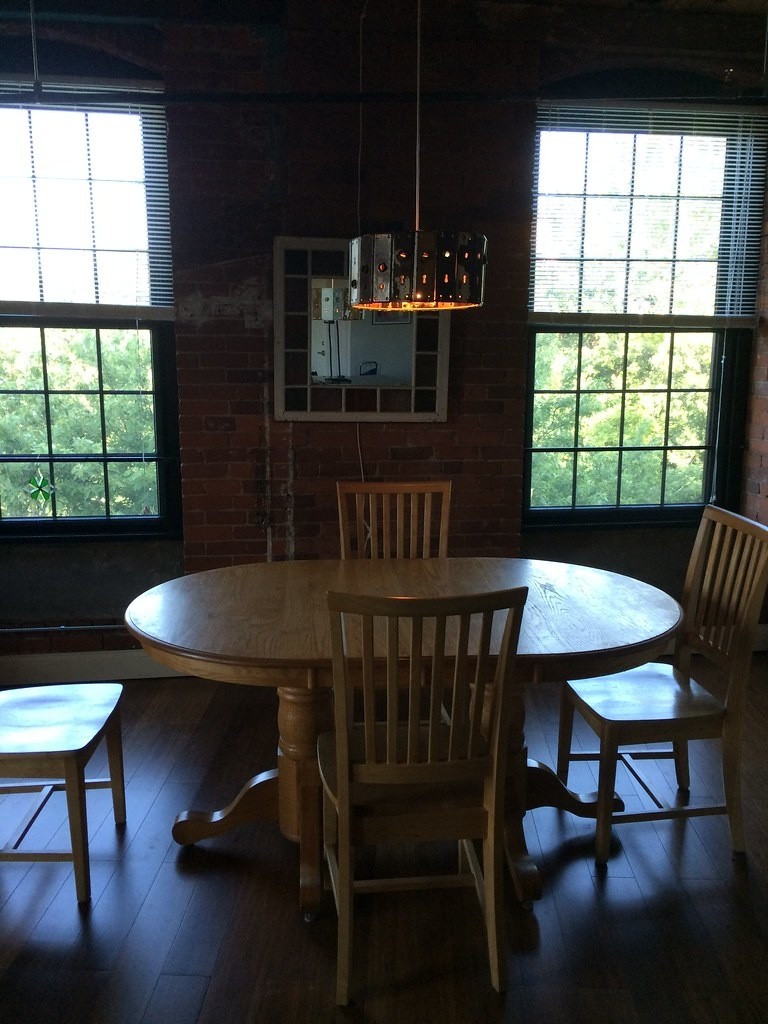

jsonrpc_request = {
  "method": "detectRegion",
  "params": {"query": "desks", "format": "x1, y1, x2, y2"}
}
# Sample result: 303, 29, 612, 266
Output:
123, 556, 686, 1003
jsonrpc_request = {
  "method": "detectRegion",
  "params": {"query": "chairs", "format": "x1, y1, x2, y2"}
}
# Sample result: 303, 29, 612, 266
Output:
1, 684, 124, 898
336, 481, 452, 557
315, 587, 531, 1004
547, 502, 768, 862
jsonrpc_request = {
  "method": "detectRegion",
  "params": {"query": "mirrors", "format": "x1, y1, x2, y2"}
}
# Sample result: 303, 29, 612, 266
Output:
308, 277, 416, 390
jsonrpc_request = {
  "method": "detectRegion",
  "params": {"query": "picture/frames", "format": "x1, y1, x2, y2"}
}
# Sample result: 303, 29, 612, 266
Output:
372, 310, 411, 325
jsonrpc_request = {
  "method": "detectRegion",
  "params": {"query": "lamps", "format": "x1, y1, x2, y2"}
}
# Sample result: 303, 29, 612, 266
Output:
311, 279, 370, 320
347, 1, 486, 314
